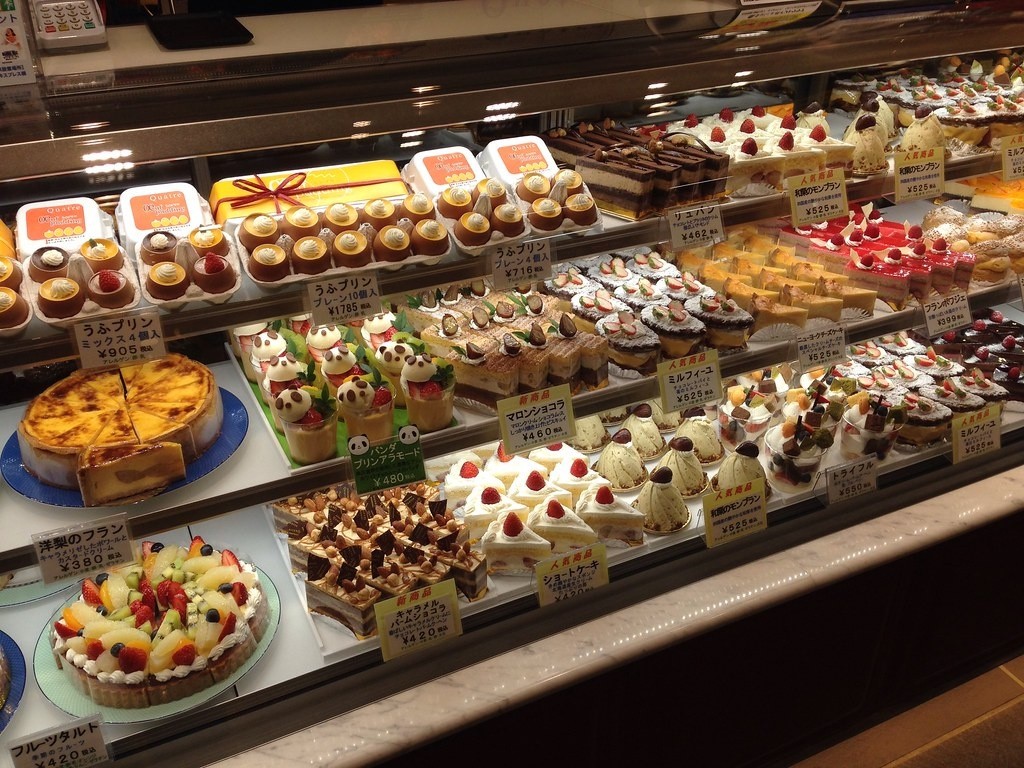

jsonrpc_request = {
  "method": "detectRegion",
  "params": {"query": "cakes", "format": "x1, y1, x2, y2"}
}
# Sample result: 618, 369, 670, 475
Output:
16, 353, 224, 490
75, 442, 187, 508
46, 532, 272, 708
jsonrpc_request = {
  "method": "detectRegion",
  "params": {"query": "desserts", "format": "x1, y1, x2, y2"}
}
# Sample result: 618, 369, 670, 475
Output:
231, 51, 1024, 643
0, 222, 237, 331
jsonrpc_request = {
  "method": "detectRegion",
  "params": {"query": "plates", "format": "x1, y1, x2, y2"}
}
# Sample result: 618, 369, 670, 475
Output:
0, 387, 249, 509
0, 629, 26, 734
0, 563, 91, 608
34, 560, 282, 726
